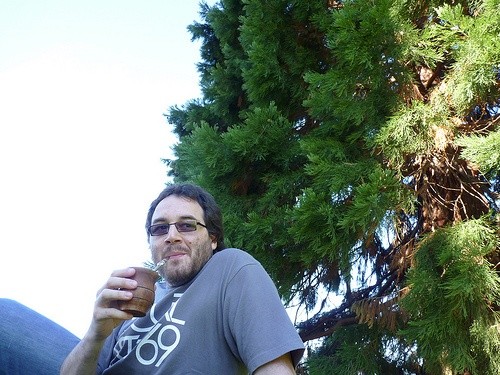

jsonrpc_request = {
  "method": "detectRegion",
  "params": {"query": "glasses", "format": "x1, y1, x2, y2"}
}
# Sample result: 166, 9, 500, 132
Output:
147, 220, 206, 236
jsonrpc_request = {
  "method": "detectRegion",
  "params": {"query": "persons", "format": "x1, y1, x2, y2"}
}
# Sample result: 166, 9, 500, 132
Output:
60, 182, 307, 375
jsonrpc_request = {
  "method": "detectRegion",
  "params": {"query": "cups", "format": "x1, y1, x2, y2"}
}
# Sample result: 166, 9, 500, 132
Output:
118, 266, 159, 318
